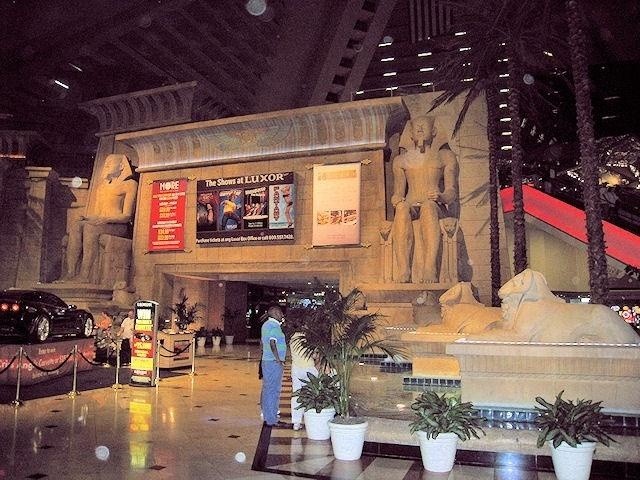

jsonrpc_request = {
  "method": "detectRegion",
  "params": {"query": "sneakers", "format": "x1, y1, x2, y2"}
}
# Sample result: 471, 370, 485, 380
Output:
260, 409, 281, 416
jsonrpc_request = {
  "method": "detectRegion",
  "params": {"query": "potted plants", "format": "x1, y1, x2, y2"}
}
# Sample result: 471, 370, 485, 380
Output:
210, 327, 224, 346
534, 389, 620, 480
290, 372, 342, 440
406, 389, 488, 473
291, 278, 413, 460
221, 307, 240, 345
195, 327, 207, 347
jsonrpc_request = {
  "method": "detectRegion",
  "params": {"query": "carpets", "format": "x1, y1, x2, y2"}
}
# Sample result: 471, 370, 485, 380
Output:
0, 364, 189, 405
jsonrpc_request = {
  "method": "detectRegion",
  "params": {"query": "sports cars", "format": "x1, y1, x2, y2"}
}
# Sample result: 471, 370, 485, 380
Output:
0, 289, 95, 343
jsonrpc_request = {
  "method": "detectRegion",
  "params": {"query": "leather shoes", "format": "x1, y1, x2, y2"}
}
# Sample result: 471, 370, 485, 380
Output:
266, 421, 285, 428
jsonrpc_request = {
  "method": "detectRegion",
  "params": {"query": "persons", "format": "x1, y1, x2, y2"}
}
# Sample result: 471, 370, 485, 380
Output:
290, 317, 322, 431
93, 309, 137, 366
282, 186, 293, 227
261, 306, 286, 426
392, 116, 459, 283
219, 193, 241, 230
204, 203, 217, 227
52, 153, 138, 284
246, 202, 267, 215
